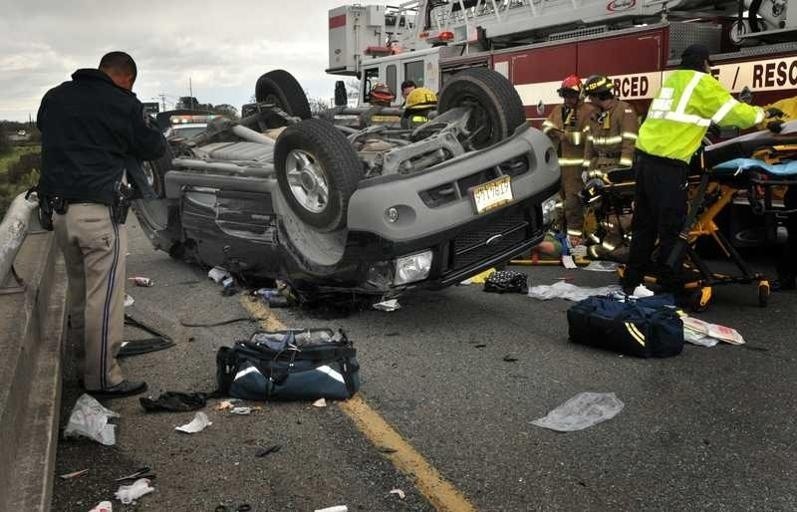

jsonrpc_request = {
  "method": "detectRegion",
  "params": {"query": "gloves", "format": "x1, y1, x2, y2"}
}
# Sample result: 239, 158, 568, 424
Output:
767, 108, 784, 118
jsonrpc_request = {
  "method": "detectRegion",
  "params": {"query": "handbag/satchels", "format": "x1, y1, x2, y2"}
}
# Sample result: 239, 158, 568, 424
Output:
481, 271, 529, 295
216, 328, 360, 400
567, 294, 684, 361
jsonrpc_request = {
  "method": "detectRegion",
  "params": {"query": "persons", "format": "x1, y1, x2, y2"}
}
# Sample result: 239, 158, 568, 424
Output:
541, 74, 640, 248
620, 44, 785, 296
401, 80, 417, 107
37, 51, 167, 402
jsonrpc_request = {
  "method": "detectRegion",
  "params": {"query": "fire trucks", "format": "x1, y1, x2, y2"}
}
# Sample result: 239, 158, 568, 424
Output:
323, 0, 797, 215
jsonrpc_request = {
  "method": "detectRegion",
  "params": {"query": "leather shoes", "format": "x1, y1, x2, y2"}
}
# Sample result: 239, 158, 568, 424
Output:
84, 379, 148, 397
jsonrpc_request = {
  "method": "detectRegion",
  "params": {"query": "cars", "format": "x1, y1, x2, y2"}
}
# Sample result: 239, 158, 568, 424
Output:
128, 69, 565, 315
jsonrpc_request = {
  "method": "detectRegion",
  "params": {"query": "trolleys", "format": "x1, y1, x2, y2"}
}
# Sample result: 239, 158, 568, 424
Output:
599, 119, 796, 310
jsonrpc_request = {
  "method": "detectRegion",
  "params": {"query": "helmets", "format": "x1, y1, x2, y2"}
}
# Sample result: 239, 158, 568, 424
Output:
402, 87, 437, 110
557, 75, 613, 100
